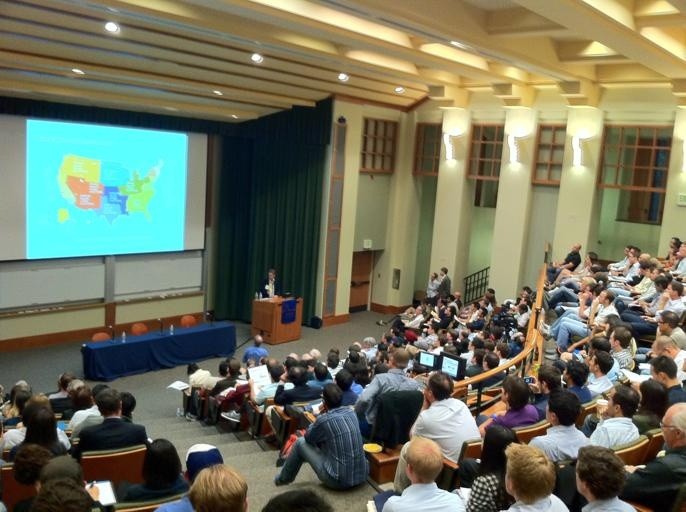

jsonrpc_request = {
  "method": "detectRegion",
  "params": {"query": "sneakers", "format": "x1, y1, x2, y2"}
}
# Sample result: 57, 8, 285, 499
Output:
539, 280, 559, 361
220, 410, 242, 423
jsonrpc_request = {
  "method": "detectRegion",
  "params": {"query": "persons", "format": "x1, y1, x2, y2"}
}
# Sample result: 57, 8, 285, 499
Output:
184, 335, 269, 427
260, 236, 685, 512
156, 443, 248, 512
1, 374, 188, 512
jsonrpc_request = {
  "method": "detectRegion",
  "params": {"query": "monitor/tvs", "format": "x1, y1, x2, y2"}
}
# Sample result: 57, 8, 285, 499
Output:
418, 350, 439, 376
439, 351, 468, 381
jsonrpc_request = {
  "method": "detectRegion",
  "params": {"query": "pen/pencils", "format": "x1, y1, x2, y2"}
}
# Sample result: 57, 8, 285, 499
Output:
90, 481, 96, 489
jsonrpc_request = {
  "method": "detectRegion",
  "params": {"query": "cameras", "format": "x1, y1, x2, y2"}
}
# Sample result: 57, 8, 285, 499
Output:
523, 376, 533, 384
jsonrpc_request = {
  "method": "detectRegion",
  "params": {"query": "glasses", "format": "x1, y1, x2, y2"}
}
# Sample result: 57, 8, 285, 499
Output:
659, 422, 675, 430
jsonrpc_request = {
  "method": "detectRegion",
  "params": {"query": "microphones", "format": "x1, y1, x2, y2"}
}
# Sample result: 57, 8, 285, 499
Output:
156, 317, 163, 335
107, 325, 117, 343
204, 312, 213, 325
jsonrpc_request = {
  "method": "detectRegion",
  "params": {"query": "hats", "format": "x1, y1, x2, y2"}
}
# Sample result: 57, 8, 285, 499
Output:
186, 443, 223, 482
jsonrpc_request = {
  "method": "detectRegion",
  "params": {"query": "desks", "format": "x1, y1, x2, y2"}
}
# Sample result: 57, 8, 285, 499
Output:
81, 322, 237, 382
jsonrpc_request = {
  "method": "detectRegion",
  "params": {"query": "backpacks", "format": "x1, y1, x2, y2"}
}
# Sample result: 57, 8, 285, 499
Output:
275, 429, 306, 466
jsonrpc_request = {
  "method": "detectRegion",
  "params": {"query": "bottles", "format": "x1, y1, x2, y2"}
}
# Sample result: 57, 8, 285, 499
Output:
259, 292, 262, 299
168, 325, 174, 336
255, 292, 258, 299
121, 331, 127, 343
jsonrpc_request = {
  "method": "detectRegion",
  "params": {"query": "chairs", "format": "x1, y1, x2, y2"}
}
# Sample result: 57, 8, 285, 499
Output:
131, 323, 148, 333
92, 333, 109, 341
180, 314, 196, 327
1, 413, 193, 512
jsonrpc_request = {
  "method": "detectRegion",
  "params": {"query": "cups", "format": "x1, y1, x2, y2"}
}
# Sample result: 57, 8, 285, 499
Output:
597, 401, 609, 416
